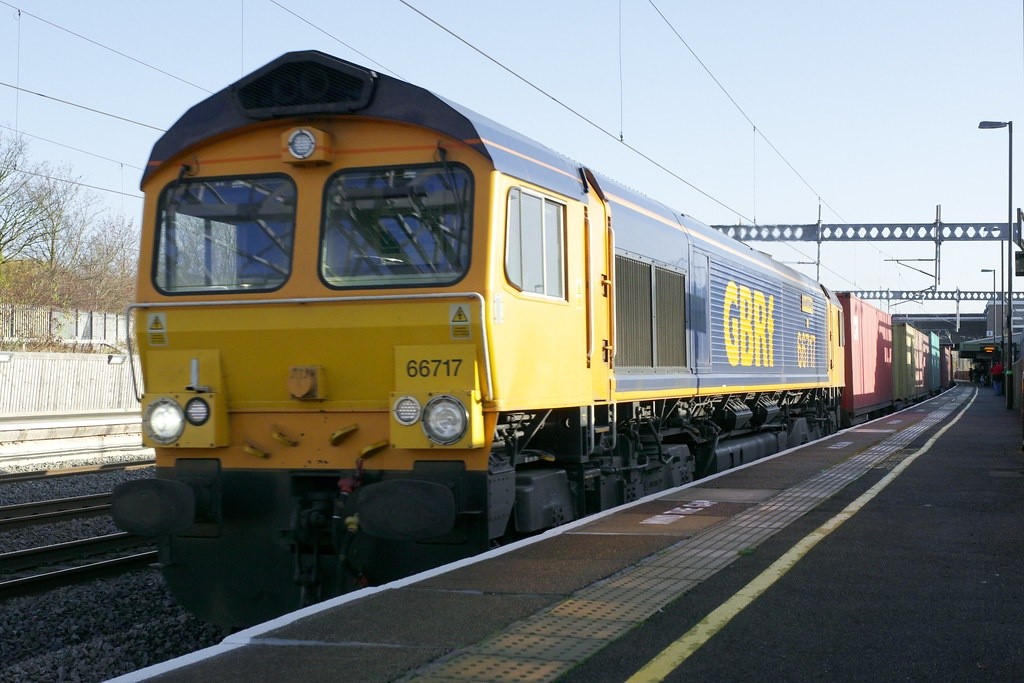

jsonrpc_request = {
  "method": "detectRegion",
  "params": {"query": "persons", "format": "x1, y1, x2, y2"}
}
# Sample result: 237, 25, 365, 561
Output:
968, 366, 986, 386
989, 359, 1004, 396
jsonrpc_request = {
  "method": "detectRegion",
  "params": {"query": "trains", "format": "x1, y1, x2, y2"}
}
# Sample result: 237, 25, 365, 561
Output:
109, 49, 956, 628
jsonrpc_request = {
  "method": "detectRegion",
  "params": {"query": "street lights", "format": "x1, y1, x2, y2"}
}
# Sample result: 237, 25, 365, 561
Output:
981, 269, 997, 364
978, 120, 1014, 410
